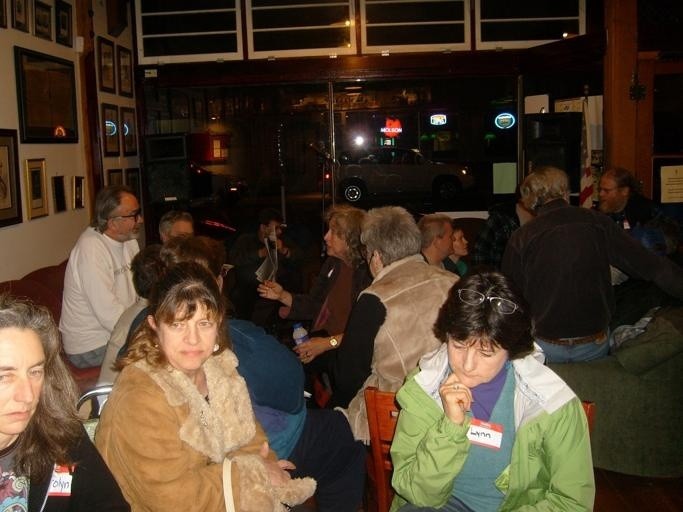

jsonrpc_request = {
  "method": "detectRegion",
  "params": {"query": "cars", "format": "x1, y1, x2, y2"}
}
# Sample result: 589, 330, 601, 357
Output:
320, 147, 468, 202
143, 160, 246, 207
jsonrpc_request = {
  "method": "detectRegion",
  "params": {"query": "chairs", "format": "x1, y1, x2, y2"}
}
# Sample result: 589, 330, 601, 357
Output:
365, 384, 597, 510
73, 385, 115, 420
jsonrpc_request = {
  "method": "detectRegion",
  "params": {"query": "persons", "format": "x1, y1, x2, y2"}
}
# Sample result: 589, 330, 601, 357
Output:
94, 260, 317, 512
0, 292, 130, 511
157, 233, 366, 512
387, 269, 595, 512
322, 206, 460, 447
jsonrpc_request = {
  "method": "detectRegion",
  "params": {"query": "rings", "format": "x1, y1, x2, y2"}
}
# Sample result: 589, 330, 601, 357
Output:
453, 383, 459, 390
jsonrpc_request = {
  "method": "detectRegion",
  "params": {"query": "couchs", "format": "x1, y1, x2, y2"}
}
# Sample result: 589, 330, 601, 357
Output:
542, 312, 683, 481
0, 255, 101, 398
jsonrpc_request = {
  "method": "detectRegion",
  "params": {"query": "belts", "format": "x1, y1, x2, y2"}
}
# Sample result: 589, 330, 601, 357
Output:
535, 331, 604, 347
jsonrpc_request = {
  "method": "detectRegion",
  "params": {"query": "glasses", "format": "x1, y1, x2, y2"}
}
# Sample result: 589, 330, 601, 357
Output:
597, 186, 615, 193
458, 288, 518, 316
111, 208, 142, 223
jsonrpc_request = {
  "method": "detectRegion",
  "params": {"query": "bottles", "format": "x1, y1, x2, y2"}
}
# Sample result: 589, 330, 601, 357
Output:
290, 324, 316, 361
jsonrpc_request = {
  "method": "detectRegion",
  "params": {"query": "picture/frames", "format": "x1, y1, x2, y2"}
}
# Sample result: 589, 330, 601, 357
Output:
1, 0, 141, 231
